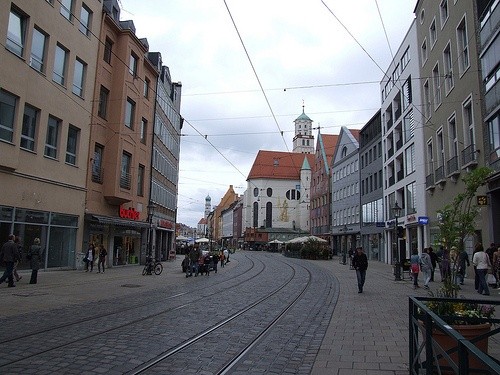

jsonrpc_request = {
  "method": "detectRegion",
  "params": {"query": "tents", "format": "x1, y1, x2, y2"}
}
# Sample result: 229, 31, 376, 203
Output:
286, 236, 308, 243
303, 236, 327, 242
269, 239, 283, 243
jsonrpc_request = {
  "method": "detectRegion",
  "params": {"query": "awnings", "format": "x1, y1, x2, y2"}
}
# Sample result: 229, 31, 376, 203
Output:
91, 214, 150, 227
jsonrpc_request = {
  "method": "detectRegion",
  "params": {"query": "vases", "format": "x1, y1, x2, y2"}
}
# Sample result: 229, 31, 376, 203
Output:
418, 321, 492, 375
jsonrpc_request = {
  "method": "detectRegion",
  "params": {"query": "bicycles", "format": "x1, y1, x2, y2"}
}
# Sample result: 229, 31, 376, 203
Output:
142, 256, 163, 275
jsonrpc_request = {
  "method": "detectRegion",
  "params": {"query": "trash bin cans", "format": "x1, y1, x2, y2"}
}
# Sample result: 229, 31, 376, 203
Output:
75, 251, 85, 270
131, 255, 136, 264
135, 257, 138, 263
169, 250, 176, 261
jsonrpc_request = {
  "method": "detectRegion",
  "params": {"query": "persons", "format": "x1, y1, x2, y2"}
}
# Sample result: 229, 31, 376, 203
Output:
351, 247, 368, 293
348, 247, 358, 256
2, 238, 22, 282
175, 242, 232, 277
96, 245, 108, 273
410, 244, 470, 290
472, 241, 500, 296
240, 242, 333, 260
83, 244, 96, 272
29, 238, 42, 284
0, 234, 17, 287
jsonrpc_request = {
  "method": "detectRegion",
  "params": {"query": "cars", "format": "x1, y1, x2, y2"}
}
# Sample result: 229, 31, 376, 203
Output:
182, 250, 214, 272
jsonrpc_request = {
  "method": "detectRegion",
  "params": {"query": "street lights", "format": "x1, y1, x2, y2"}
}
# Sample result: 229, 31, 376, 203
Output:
210, 224, 214, 256
392, 200, 401, 281
343, 225, 348, 265
146, 201, 157, 275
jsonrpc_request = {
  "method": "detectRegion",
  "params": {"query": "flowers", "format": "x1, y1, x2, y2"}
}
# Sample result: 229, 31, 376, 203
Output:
418, 167, 495, 325
